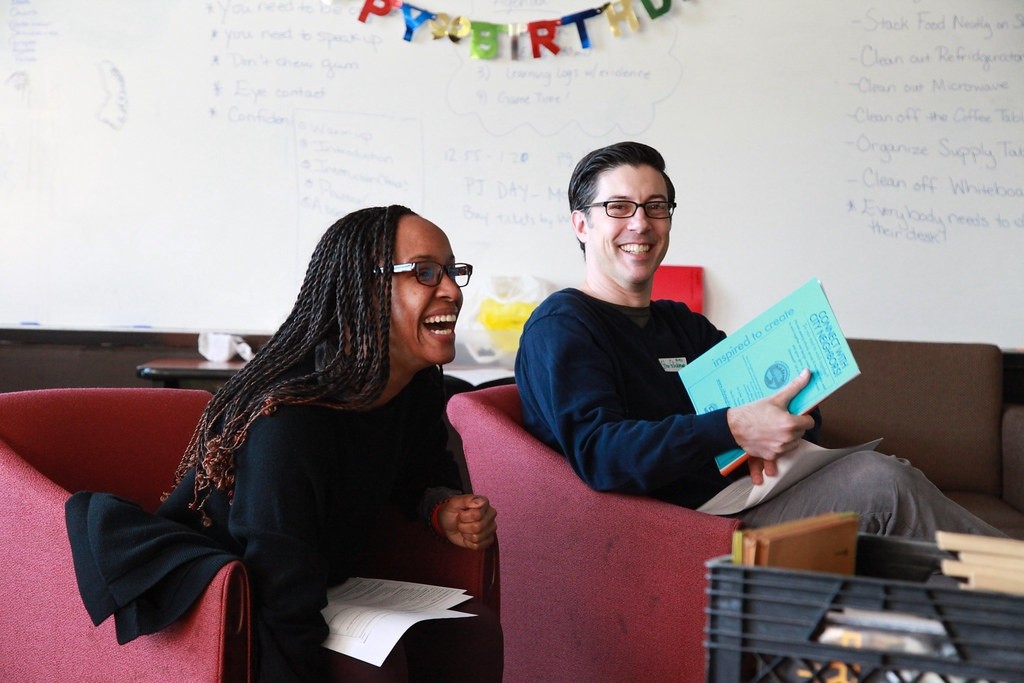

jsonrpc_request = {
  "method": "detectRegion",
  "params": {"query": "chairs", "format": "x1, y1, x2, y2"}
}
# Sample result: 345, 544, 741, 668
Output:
0, 388, 253, 683
812, 336, 1024, 547
446, 384, 748, 683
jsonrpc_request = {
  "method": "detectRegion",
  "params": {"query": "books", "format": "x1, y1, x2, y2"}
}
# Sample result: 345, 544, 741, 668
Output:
824, 605, 987, 683
935, 530, 1024, 598
730, 509, 862, 683
678, 279, 862, 475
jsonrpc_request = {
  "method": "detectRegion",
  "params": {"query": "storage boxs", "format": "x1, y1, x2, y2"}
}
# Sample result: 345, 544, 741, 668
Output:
697, 526, 1024, 683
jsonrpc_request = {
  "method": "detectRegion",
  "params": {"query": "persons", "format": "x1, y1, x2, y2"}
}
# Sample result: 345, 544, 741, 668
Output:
63, 204, 496, 683
514, 141, 1011, 541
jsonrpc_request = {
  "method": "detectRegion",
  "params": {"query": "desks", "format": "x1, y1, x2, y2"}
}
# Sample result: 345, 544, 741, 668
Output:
135, 355, 248, 391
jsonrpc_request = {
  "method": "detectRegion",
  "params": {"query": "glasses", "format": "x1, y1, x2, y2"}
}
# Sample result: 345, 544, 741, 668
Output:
584, 200, 676, 220
371, 261, 473, 287
